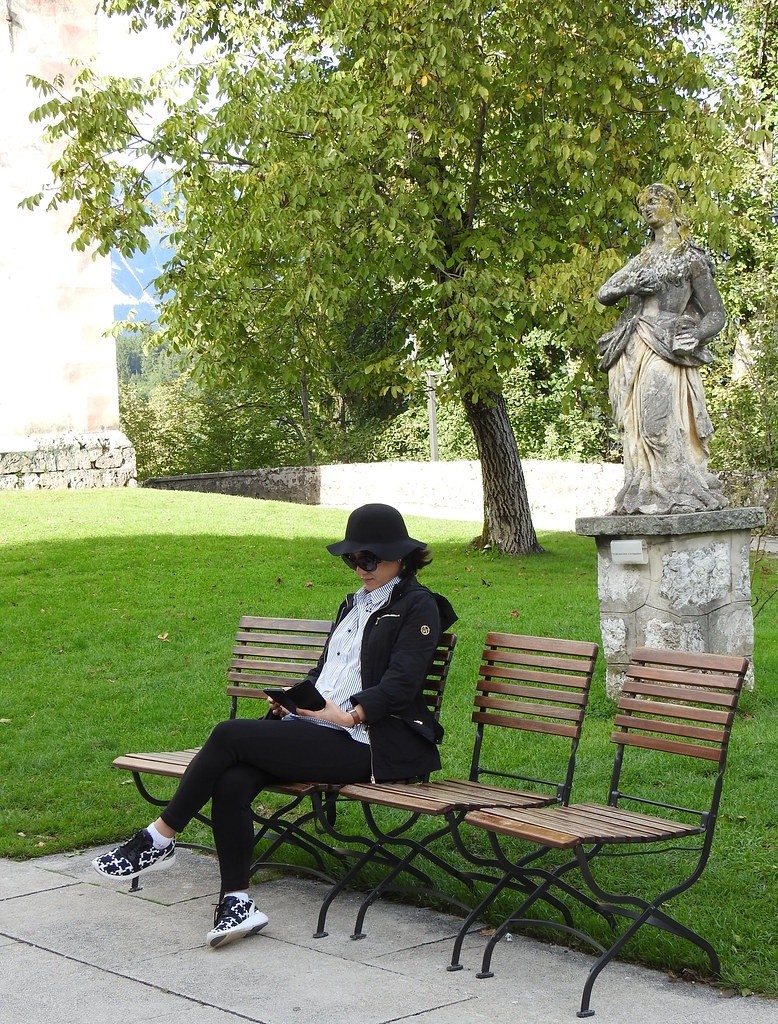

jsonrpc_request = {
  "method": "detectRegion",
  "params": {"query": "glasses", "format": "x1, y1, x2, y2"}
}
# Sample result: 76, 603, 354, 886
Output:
342, 552, 386, 571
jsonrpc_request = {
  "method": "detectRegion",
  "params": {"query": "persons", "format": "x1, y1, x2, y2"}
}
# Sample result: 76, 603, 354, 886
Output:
91, 503, 458, 948
598, 183, 728, 515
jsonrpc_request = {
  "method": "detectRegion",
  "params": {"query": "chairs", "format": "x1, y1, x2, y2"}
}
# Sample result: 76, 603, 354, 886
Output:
113, 615, 748, 1019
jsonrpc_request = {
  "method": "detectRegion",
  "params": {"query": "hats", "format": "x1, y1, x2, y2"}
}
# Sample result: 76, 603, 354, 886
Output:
326, 504, 428, 561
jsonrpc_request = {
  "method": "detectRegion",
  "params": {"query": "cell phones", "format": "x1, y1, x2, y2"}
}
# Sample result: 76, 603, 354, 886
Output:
264, 680, 326, 716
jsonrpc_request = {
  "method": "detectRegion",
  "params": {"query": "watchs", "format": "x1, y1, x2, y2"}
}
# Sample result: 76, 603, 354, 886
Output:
346, 707, 362, 729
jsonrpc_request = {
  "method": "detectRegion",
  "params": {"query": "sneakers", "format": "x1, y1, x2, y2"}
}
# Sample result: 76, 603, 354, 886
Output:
206, 894, 270, 946
91, 828, 176, 881
